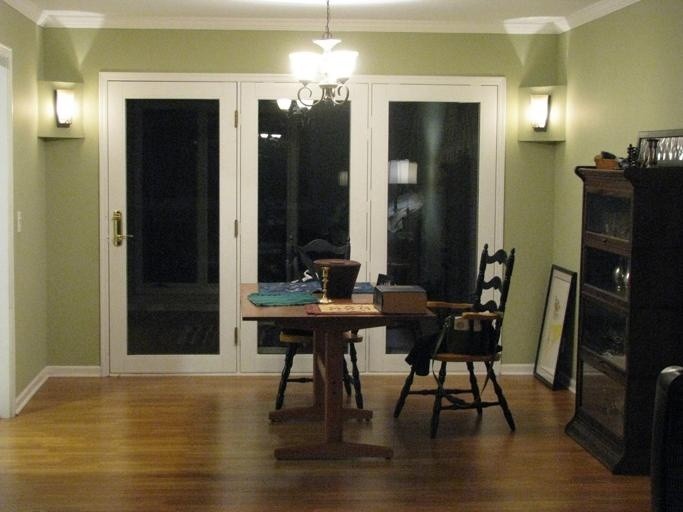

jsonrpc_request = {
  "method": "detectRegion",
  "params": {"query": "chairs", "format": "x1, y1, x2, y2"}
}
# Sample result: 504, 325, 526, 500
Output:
275, 233, 362, 410
394, 243, 516, 438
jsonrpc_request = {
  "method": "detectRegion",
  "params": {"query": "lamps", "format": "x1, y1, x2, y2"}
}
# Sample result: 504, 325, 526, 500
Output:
277, 99, 315, 129
530, 94, 551, 132
287, 1, 360, 107
55, 88, 73, 127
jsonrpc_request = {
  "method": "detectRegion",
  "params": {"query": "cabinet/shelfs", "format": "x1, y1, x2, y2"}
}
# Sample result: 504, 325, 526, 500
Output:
564, 166, 683, 475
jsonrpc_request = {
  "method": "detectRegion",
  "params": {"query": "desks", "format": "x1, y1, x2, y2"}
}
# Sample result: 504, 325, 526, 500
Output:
240, 283, 437, 460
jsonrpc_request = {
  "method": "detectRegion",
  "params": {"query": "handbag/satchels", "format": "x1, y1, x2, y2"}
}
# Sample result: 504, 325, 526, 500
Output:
443, 314, 497, 355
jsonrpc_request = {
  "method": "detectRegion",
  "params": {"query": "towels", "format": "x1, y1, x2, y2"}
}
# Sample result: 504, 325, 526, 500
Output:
248, 291, 319, 307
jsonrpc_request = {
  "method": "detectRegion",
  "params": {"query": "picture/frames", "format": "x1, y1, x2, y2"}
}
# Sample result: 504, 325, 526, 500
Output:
533, 263, 577, 389
